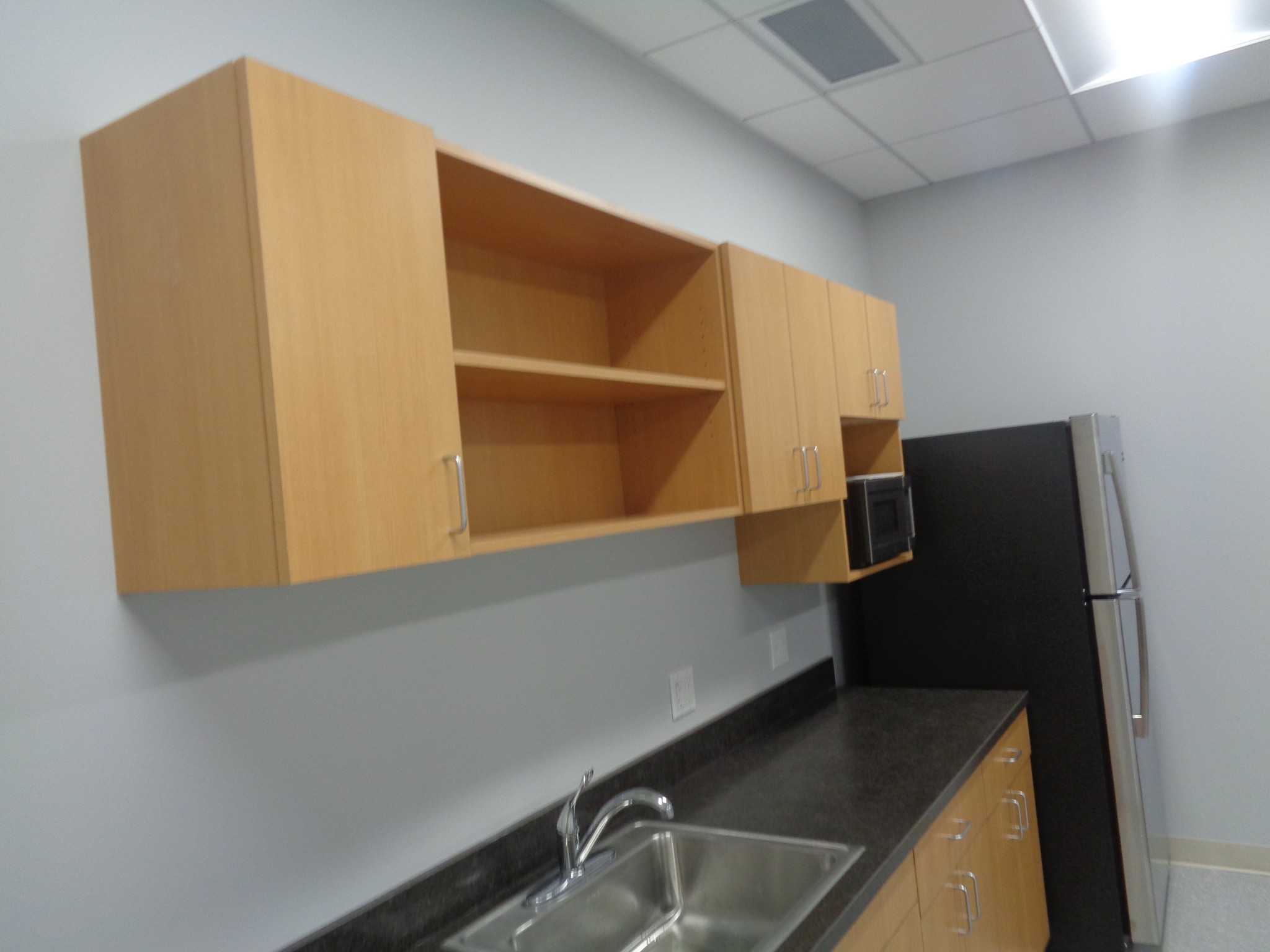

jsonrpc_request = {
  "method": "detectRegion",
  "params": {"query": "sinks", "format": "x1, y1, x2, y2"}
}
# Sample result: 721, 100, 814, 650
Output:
439, 817, 867, 952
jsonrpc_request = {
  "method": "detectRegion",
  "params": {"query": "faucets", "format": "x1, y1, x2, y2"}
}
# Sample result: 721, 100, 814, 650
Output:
552, 767, 675, 877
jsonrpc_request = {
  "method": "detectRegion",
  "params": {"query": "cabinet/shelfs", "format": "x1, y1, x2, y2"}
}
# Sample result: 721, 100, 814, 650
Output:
78, 57, 469, 592
719, 242, 846, 512
828, 280, 904, 420
434, 138, 745, 556
834, 709, 1049, 952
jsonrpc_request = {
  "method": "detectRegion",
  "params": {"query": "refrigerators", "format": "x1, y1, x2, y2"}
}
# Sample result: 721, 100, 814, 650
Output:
838, 412, 1168, 952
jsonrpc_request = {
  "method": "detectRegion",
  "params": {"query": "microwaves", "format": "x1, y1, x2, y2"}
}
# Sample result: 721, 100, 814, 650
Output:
843, 471, 916, 570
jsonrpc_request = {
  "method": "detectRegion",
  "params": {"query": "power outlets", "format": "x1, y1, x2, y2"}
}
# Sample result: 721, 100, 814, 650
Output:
668, 666, 696, 721
769, 629, 789, 671
